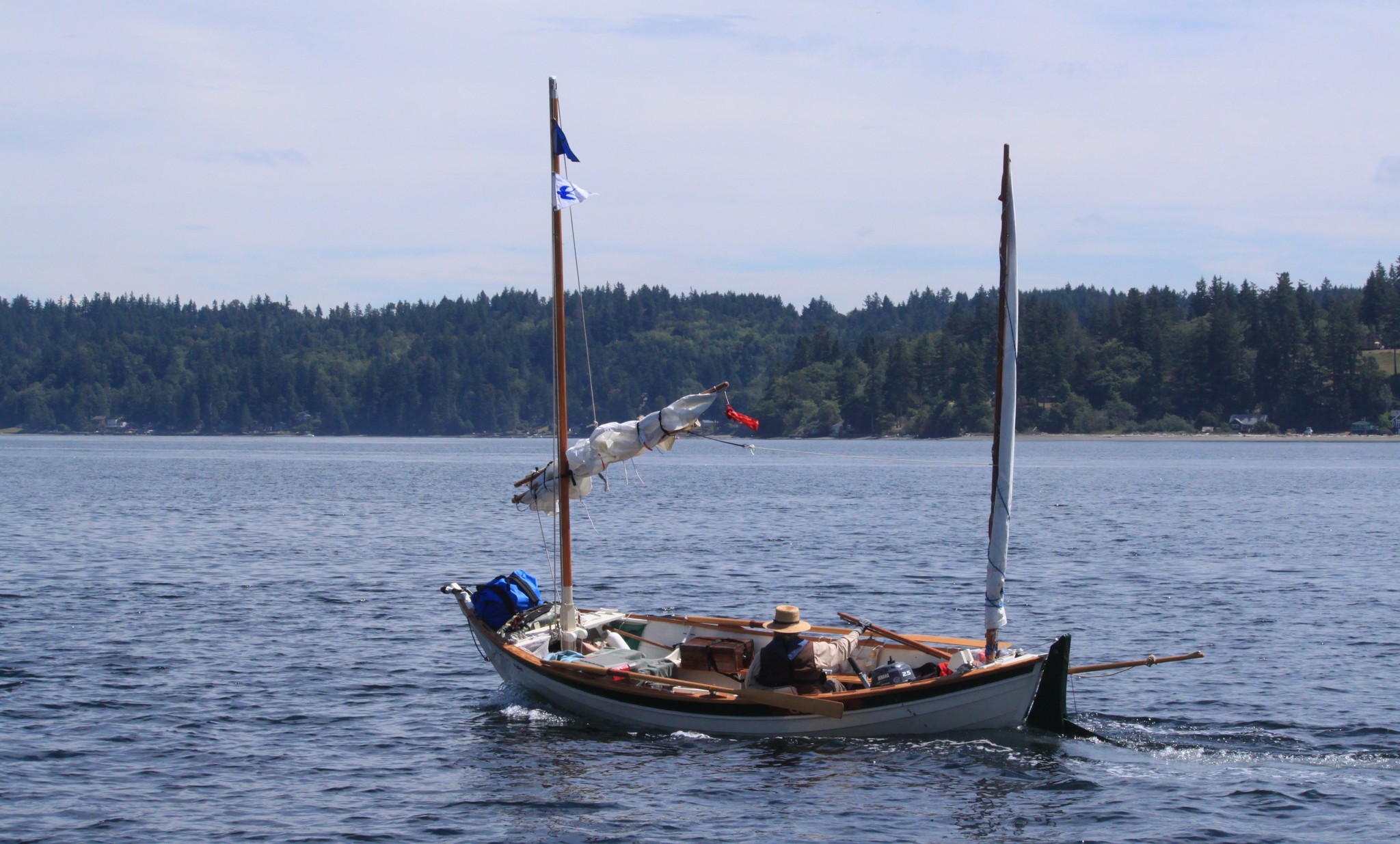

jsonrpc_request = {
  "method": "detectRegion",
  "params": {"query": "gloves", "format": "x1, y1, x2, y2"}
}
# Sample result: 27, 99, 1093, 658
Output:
852, 617, 872, 636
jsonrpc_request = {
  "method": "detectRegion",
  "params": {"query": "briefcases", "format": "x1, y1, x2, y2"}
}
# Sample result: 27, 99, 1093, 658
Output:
679, 636, 754, 673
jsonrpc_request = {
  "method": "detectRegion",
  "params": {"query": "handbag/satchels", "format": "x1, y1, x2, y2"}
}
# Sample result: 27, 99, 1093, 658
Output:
470, 570, 543, 632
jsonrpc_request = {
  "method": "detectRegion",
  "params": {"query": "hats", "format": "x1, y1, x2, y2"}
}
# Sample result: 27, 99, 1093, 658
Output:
762, 605, 811, 633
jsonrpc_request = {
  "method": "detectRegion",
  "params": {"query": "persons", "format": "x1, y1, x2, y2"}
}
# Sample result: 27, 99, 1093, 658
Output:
744, 605, 873, 694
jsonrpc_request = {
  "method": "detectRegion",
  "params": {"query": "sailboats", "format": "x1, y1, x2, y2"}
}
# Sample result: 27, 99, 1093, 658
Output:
442, 73, 1046, 736
